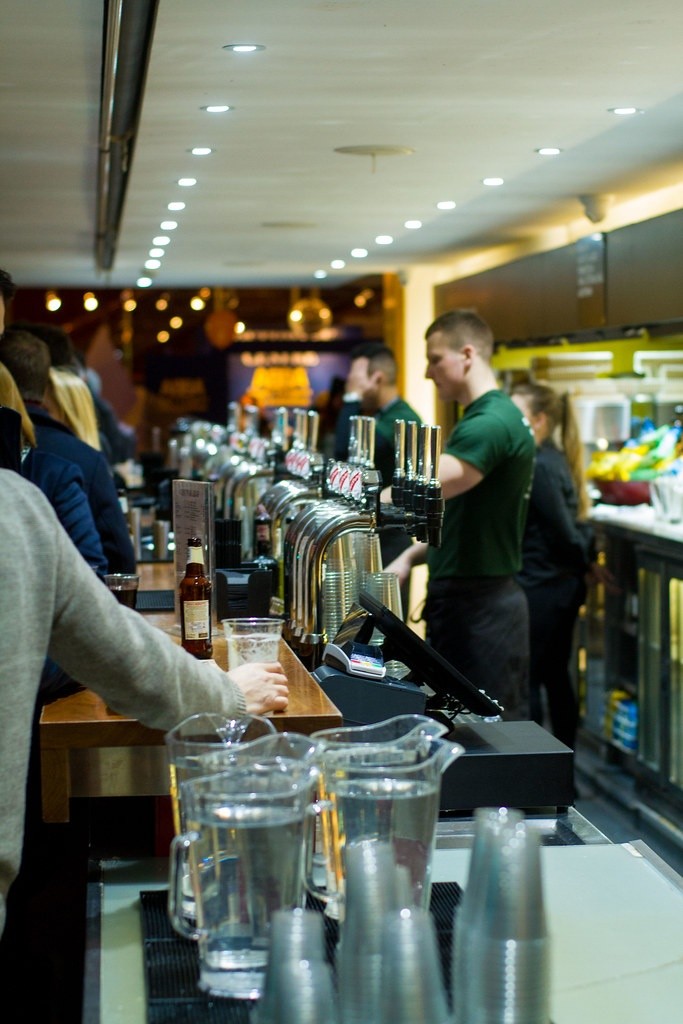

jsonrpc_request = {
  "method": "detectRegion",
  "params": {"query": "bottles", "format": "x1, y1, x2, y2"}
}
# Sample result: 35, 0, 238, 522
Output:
178, 537, 214, 660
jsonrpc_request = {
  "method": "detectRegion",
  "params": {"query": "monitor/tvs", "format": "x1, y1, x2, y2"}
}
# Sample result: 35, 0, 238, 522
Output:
357, 588, 502, 726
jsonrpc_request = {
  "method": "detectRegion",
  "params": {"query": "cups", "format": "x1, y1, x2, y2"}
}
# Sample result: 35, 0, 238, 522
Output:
104, 572, 140, 609
321, 532, 405, 648
165, 708, 551, 1024
221, 617, 285, 671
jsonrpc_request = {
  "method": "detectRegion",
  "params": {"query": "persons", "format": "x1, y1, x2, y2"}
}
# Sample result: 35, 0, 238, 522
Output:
509, 380, 591, 801
0, 269, 289, 1024
334, 343, 424, 625
376, 311, 540, 719
